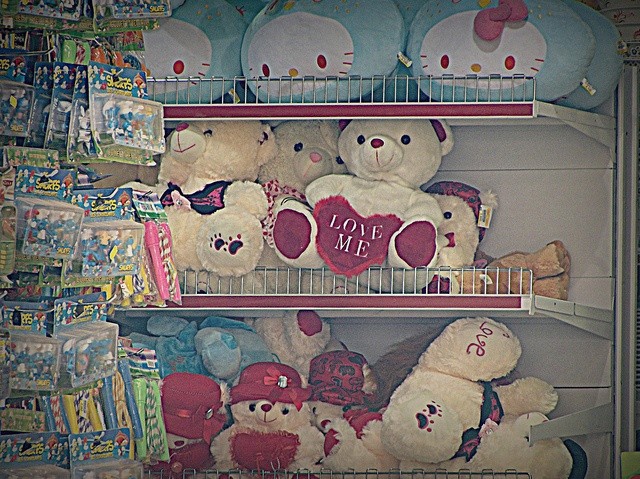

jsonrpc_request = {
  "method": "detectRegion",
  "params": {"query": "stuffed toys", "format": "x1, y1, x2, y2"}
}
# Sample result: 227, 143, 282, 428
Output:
307, 351, 399, 479
299, 274, 375, 297
551, 1, 628, 109
240, 2, 407, 103
127, 312, 273, 379
406, 1, 596, 100
382, 317, 573, 479
150, 372, 232, 479
136, 0, 247, 105
257, 120, 349, 274
241, 309, 330, 377
461, 239, 571, 300
154, 119, 278, 279
210, 363, 325, 478
175, 266, 300, 295
423, 182, 499, 295
305, 117, 454, 292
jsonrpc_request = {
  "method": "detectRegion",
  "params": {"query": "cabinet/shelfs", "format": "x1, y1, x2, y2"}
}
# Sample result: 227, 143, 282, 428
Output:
0, 0, 186, 479
133, 1, 640, 479
